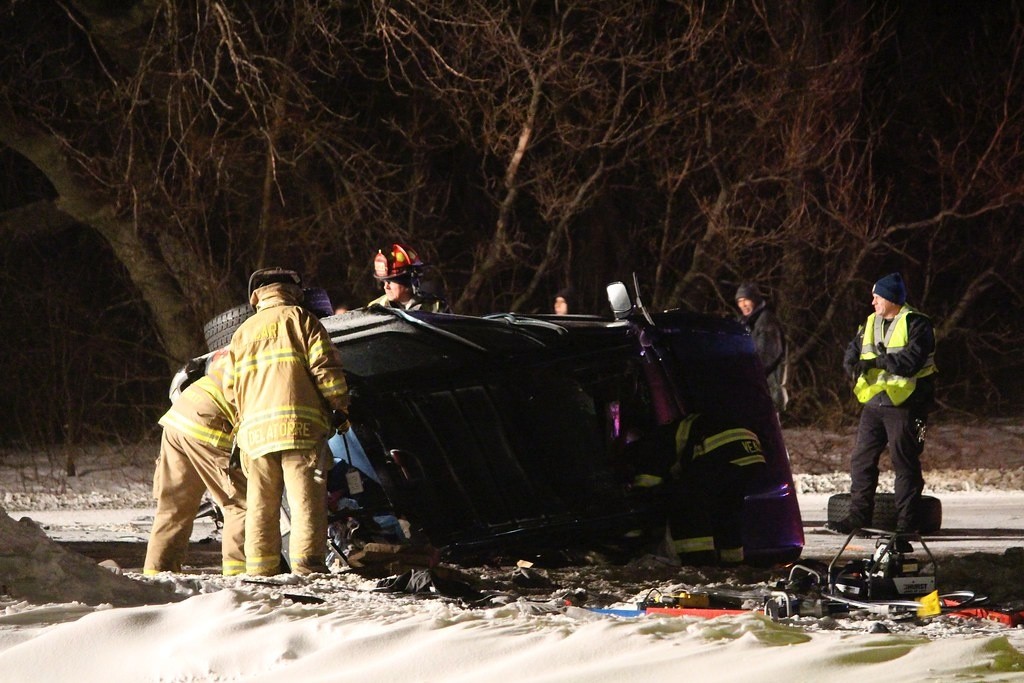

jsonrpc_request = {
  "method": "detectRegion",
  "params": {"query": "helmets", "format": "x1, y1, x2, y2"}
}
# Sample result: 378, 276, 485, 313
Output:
248, 268, 305, 314
374, 245, 424, 280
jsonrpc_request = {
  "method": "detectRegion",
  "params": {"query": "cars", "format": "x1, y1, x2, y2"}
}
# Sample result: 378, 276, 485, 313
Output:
201, 286, 808, 582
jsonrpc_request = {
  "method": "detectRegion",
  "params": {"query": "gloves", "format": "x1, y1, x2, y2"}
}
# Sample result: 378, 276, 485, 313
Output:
854, 358, 877, 376
333, 409, 350, 436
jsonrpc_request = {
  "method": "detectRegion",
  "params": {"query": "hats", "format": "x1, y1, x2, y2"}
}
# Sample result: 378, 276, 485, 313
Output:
873, 274, 906, 306
734, 283, 762, 310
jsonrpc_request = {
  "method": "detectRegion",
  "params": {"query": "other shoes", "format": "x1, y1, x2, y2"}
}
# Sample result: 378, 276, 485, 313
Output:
893, 523, 918, 535
824, 518, 872, 539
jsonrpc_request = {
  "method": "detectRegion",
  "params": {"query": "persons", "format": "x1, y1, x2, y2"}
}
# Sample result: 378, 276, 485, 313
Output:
366, 244, 457, 311
335, 302, 352, 314
822, 271, 935, 540
553, 288, 581, 313
143, 346, 248, 578
218, 270, 351, 576
736, 281, 788, 423
